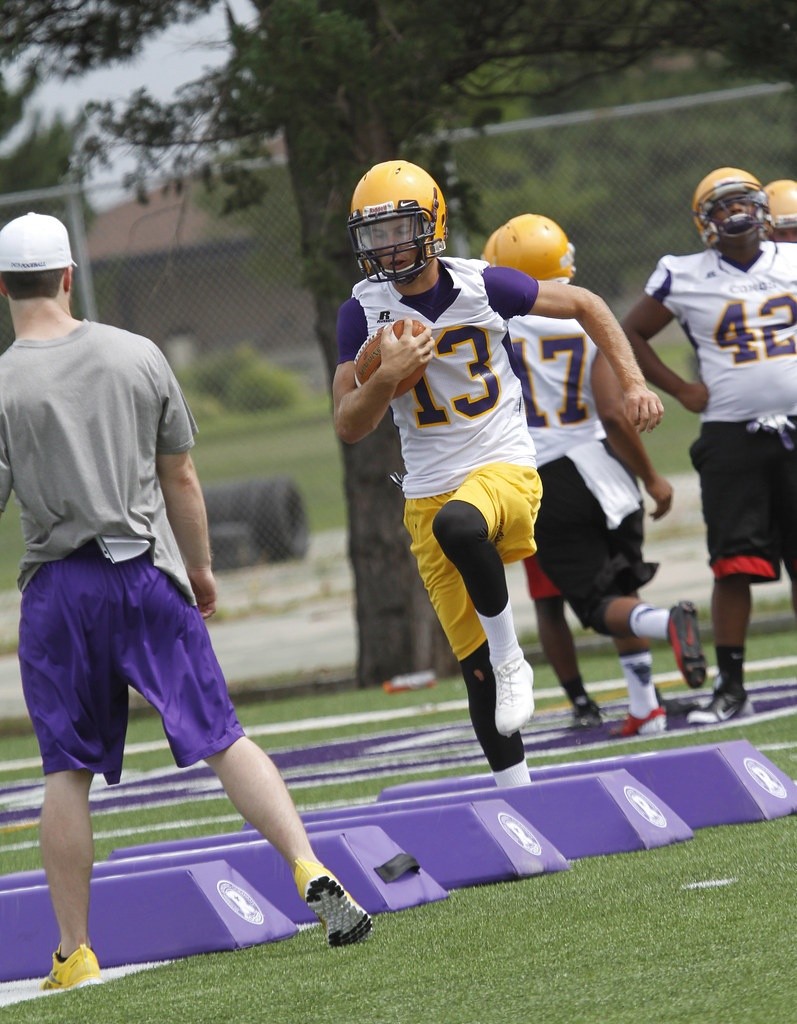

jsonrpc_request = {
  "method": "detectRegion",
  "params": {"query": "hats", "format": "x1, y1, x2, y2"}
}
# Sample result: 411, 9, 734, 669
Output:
0, 212, 77, 272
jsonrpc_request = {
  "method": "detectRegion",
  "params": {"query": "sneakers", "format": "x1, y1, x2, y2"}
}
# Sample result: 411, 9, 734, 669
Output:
685, 685, 756, 726
492, 653, 534, 733
609, 707, 667, 738
667, 600, 706, 688
40, 945, 103, 990
294, 859, 372, 945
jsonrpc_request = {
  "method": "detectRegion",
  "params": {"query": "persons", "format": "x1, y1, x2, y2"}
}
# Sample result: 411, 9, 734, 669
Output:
622, 166, 797, 724
1, 212, 374, 991
762, 179, 797, 242
333, 159, 664, 795
476, 215, 706, 738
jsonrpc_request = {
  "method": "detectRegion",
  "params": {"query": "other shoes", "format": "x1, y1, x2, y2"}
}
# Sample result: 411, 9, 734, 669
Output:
570, 707, 600, 733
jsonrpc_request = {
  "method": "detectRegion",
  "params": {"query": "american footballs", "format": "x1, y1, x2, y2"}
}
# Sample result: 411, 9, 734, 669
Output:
352, 318, 432, 401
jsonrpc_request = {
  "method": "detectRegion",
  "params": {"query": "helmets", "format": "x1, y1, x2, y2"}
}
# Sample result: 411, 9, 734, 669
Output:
347, 160, 449, 286
480, 214, 576, 282
763, 180, 797, 238
691, 167, 773, 245
